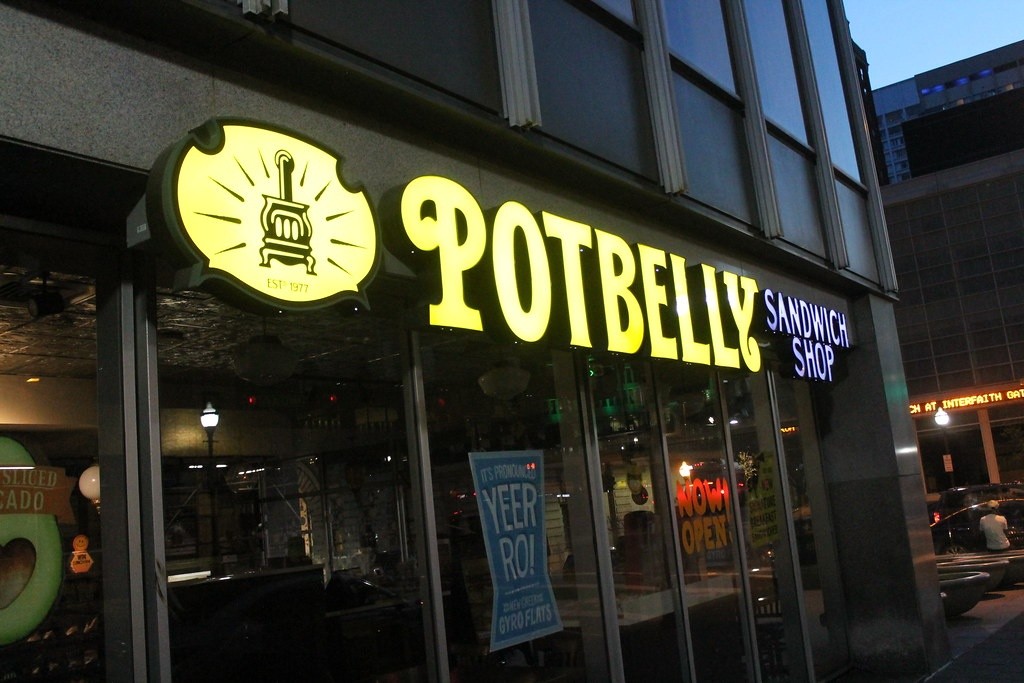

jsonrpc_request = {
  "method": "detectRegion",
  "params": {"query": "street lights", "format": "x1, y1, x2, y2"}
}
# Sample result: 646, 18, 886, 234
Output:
935, 407, 954, 487
199, 402, 227, 578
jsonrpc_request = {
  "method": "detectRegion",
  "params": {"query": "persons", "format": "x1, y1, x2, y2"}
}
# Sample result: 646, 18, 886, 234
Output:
219, 530, 239, 552
165, 525, 190, 554
976, 500, 1010, 551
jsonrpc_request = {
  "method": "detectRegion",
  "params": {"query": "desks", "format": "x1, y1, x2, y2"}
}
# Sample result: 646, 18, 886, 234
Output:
164, 563, 325, 589
321, 588, 451, 617
562, 575, 737, 634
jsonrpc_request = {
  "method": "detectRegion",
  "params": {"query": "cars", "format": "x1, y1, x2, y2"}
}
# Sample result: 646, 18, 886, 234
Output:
929, 480, 1024, 554
690, 458, 756, 491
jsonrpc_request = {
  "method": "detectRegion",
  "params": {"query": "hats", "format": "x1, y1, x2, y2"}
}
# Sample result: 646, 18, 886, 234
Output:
986, 500, 1000, 510
